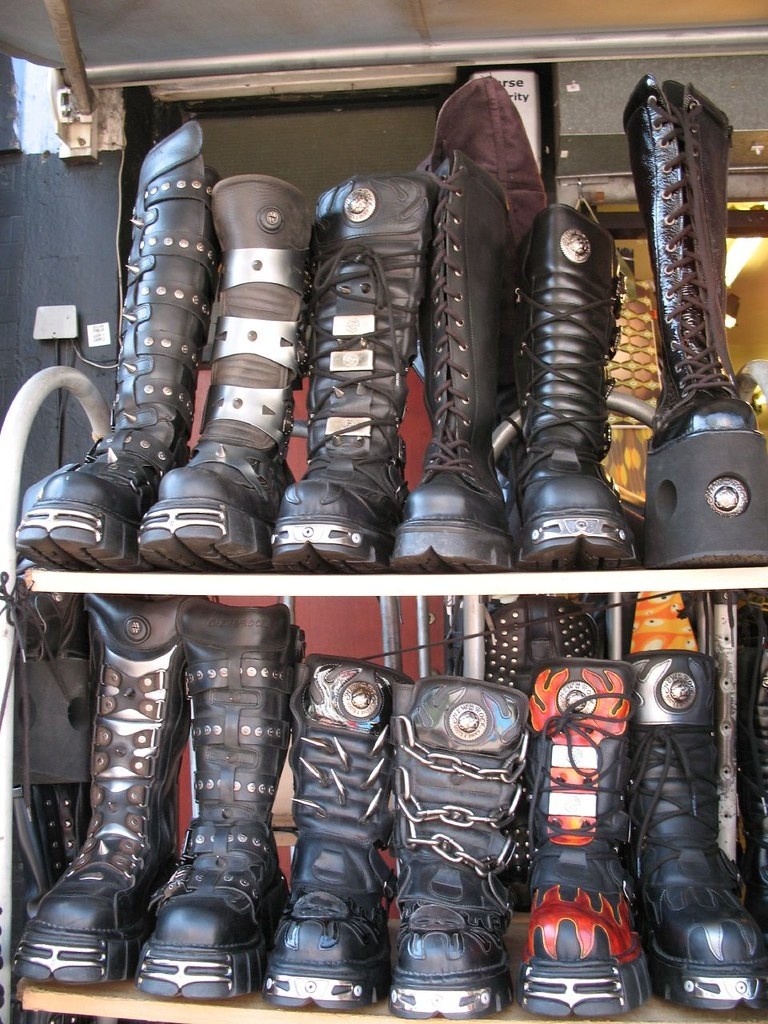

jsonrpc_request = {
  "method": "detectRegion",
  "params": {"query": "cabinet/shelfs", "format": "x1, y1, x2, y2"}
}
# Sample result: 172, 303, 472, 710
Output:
15, 567, 768, 1023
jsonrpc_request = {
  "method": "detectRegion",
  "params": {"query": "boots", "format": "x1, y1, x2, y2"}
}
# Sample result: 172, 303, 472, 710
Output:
519, 655, 651, 1020
139, 169, 315, 579
377, 675, 530, 1021
13, 594, 211, 988
0, 571, 97, 920
16, 121, 223, 571
387, 147, 516, 573
623, 74, 768, 567
510, 205, 638, 568
135, 597, 307, 1003
623, 648, 768, 1014
272, 171, 438, 574
261, 652, 413, 1014
